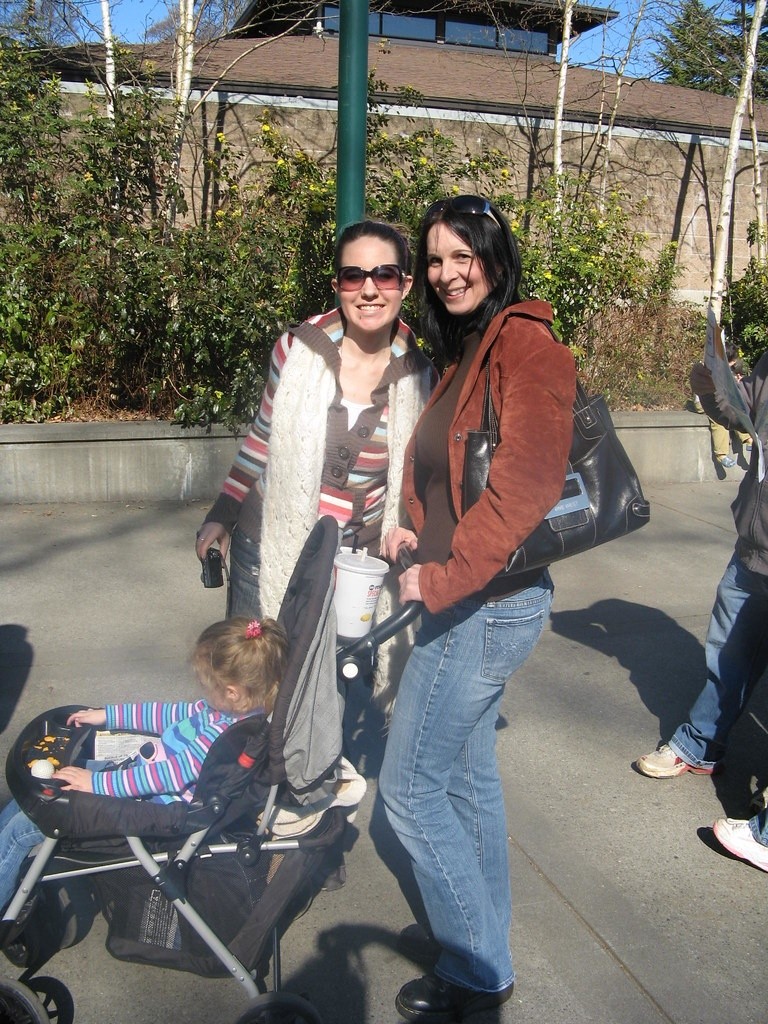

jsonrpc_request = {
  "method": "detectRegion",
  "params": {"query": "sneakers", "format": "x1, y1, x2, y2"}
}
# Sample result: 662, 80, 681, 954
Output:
713, 818, 768, 872
636, 742, 726, 779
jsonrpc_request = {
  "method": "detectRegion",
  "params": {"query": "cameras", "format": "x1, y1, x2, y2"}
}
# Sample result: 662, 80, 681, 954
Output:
199, 547, 225, 589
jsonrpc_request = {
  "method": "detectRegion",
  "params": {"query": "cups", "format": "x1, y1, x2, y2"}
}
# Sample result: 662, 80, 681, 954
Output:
332, 553, 389, 639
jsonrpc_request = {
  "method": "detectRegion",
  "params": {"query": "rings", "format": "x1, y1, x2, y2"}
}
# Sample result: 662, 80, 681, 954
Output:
199, 537, 206, 541
196, 531, 200, 539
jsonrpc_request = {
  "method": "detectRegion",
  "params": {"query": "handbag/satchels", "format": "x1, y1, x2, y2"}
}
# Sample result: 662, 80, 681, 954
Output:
461, 314, 652, 579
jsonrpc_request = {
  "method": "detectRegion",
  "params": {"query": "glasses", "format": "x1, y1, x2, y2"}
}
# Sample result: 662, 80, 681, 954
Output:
333, 264, 404, 292
423, 194, 502, 232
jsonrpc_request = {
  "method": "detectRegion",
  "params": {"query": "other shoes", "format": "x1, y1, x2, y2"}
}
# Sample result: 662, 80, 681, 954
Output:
714, 454, 735, 468
746, 439, 752, 451
398, 924, 443, 963
395, 974, 513, 1024
310, 833, 345, 891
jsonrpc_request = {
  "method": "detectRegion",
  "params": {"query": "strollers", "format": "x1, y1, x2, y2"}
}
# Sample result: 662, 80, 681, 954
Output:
0, 513, 425, 1024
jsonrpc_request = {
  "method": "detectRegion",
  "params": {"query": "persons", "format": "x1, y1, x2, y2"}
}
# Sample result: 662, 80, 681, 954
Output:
379, 195, 575, 1023
195, 219, 440, 892
0, 615, 288, 914
637, 340, 768, 873
